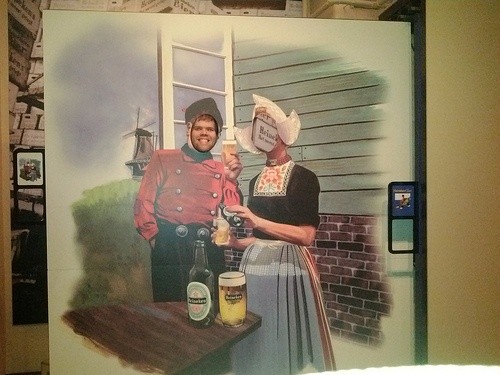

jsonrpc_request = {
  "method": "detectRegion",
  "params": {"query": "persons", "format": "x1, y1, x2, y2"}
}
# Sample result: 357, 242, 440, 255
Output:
191, 113, 219, 153
